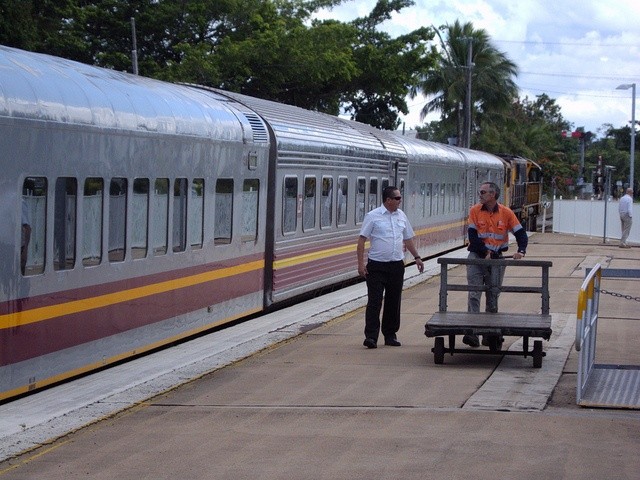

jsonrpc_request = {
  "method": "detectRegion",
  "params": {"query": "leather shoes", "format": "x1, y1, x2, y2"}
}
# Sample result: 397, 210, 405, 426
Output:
364, 337, 377, 348
385, 339, 401, 346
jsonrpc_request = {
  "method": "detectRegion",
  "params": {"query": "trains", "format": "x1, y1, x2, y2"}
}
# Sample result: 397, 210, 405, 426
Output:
0, 45, 528, 406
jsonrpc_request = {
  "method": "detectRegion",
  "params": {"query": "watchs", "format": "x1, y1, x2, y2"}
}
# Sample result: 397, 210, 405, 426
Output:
415, 256, 421, 260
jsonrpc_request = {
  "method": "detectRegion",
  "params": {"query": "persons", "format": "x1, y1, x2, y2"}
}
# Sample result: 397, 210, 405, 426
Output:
619, 188, 633, 249
357, 186, 424, 349
463, 181, 528, 347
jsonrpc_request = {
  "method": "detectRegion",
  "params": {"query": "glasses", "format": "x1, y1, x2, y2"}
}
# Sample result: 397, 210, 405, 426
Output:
480, 190, 491, 194
393, 196, 401, 200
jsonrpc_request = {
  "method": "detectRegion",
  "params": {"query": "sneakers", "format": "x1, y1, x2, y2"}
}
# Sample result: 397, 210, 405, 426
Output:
463, 334, 480, 347
483, 339, 490, 346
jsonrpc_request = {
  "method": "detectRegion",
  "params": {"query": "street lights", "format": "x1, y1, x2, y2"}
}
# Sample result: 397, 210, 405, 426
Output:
615, 83, 638, 210
